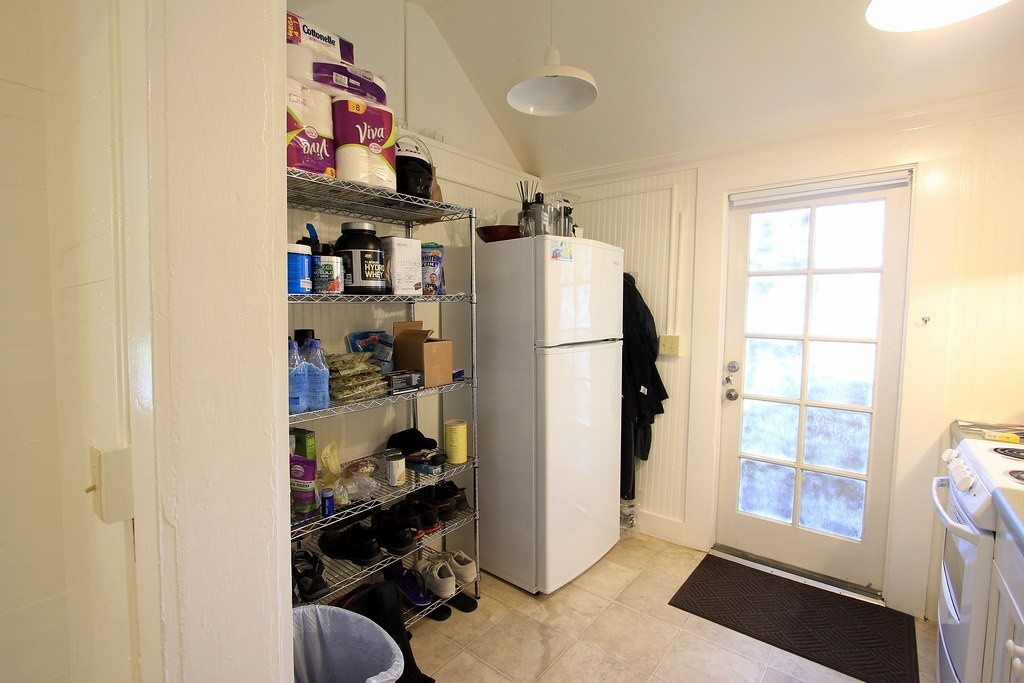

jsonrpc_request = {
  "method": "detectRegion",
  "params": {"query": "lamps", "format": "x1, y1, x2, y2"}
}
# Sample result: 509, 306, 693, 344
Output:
865, 0, 1015, 32
507, 0, 597, 118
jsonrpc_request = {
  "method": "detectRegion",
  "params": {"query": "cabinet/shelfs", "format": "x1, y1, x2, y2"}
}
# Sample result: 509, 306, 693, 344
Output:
288, 167, 481, 630
980, 515, 1024, 683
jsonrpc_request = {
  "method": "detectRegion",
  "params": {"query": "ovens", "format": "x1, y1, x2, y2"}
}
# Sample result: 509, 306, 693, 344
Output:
932, 476, 996, 683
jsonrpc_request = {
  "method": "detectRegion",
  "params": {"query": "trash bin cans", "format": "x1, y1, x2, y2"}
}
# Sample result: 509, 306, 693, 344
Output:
293, 603, 405, 683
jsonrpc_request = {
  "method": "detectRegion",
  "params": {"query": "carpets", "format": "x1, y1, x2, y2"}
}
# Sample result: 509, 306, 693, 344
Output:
669, 554, 920, 683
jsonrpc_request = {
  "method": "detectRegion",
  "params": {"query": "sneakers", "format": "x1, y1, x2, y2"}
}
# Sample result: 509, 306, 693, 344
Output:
392, 500, 440, 533
431, 481, 469, 512
415, 559, 455, 598
389, 506, 425, 540
371, 510, 417, 555
428, 549, 477, 583
406, 487, 458, 521
317, 522, 383, 566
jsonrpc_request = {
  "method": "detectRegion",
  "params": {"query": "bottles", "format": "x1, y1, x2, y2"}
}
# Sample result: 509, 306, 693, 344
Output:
288, 338, 330, 415
532, 192, 549, 236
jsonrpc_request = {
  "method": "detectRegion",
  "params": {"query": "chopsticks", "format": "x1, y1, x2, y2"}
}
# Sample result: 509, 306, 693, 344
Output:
516, 180, 538, 203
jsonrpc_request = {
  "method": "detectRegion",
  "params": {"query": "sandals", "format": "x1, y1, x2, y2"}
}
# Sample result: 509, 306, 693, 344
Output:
292, 548, 327, 597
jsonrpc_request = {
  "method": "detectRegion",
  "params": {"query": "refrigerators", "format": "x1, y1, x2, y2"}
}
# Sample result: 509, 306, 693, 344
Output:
440, 234, 624, 596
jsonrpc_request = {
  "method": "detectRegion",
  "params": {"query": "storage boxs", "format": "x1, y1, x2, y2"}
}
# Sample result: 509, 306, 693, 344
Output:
381, 237, 424, 295
452, 368, 464, 380
289, 428, 316, 461
386, 370, 424, 394
393, 322, 453, 388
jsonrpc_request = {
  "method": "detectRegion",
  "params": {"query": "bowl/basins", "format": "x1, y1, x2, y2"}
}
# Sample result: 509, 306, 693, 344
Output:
476, 225, 519, 243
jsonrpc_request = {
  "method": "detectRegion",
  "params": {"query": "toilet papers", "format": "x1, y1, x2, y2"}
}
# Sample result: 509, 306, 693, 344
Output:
287, 76, 396, 194
287, 44, 387, 104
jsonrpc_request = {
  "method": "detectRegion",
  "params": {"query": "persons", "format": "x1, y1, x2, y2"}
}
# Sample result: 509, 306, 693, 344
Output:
328, 276, 341, 291
424, 273, 437, 295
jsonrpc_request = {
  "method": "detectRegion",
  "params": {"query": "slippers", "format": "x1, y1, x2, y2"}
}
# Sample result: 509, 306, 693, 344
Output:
416, 602, 452, 620
394, 567, 431, 606
437, 586, 479, 613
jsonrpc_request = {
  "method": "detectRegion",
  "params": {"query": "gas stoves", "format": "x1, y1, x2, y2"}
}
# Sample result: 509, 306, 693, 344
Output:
943, 438, 1024, 529
